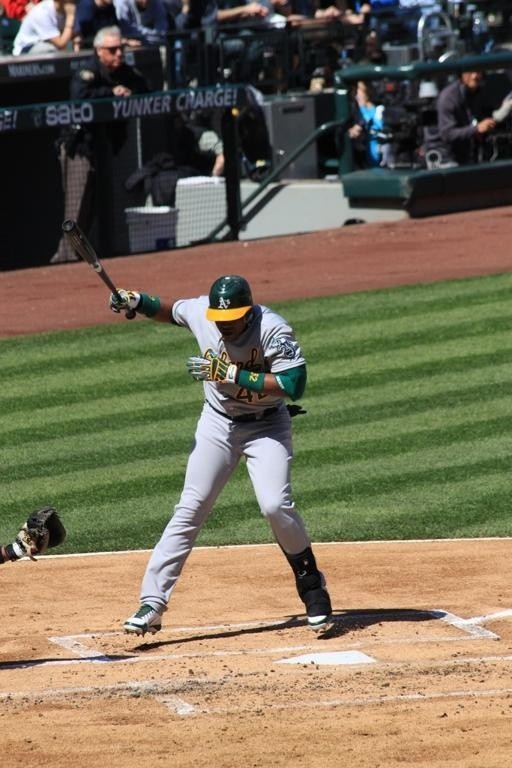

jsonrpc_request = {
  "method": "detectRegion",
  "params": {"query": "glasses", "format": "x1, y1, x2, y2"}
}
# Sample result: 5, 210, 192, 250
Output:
100, 44, 126, 54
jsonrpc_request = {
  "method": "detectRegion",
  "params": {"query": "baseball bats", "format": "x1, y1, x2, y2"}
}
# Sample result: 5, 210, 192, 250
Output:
62, 220, 135, 318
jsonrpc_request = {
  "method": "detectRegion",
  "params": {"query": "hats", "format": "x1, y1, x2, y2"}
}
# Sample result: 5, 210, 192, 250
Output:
203, 274, 255, 323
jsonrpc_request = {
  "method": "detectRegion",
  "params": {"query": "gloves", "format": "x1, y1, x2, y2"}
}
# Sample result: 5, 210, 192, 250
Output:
184, 348, 239, 386
105, 286, 142, 313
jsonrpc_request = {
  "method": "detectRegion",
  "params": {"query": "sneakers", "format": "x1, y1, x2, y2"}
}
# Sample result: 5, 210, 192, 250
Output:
295, 570, 334, 626
123, 604, 164, 638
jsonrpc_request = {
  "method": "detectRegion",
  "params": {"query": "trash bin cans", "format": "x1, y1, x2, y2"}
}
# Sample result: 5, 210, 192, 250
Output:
123, 206, 179, 254
261, 97, 319, 179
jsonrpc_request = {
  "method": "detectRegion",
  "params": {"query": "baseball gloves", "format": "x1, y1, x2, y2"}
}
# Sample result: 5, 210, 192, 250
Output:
16, 506, 66, 555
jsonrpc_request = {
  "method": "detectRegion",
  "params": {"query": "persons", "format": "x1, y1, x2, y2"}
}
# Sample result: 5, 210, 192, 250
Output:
1, 507, 68, 569
2, 1, 512, 211
106, 270, 335, 633
